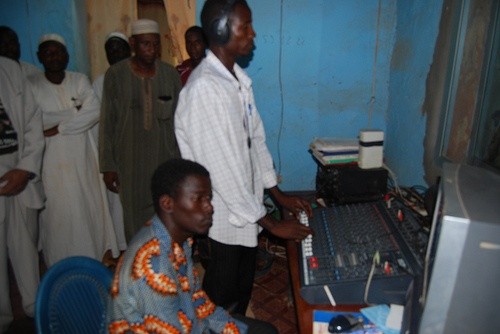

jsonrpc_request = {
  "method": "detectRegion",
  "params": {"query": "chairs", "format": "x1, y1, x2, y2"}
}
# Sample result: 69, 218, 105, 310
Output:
35, 252, 116, 334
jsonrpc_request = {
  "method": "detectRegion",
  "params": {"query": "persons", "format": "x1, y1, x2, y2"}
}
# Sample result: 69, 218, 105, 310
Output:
0, 25, 42, 81
174, 0, 315, 318
37, 33, 128, 270
105, 160, 280, 334
0, 57, 46, 334
93, 18, 205, 247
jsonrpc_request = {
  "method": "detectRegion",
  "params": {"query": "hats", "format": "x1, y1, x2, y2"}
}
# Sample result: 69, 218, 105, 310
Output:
106, 32, 129, 43
132, 20, 159, 34
39, 33, 65, 48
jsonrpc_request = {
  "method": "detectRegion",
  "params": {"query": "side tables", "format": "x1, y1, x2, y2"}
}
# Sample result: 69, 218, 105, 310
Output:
312, 158, 389, 195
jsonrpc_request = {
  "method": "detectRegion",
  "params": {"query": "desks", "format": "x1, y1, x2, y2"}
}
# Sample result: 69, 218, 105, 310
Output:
277, 191, 369, 334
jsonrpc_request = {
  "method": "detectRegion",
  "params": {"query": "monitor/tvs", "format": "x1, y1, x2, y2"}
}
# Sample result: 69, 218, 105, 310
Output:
415, 162, 500, 334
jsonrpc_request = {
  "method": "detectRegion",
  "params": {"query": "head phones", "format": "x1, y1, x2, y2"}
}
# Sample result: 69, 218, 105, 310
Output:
208, 0, 236, 44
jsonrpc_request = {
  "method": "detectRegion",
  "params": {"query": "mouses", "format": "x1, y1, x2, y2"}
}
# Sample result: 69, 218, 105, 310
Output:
328, 314, 360, 333
